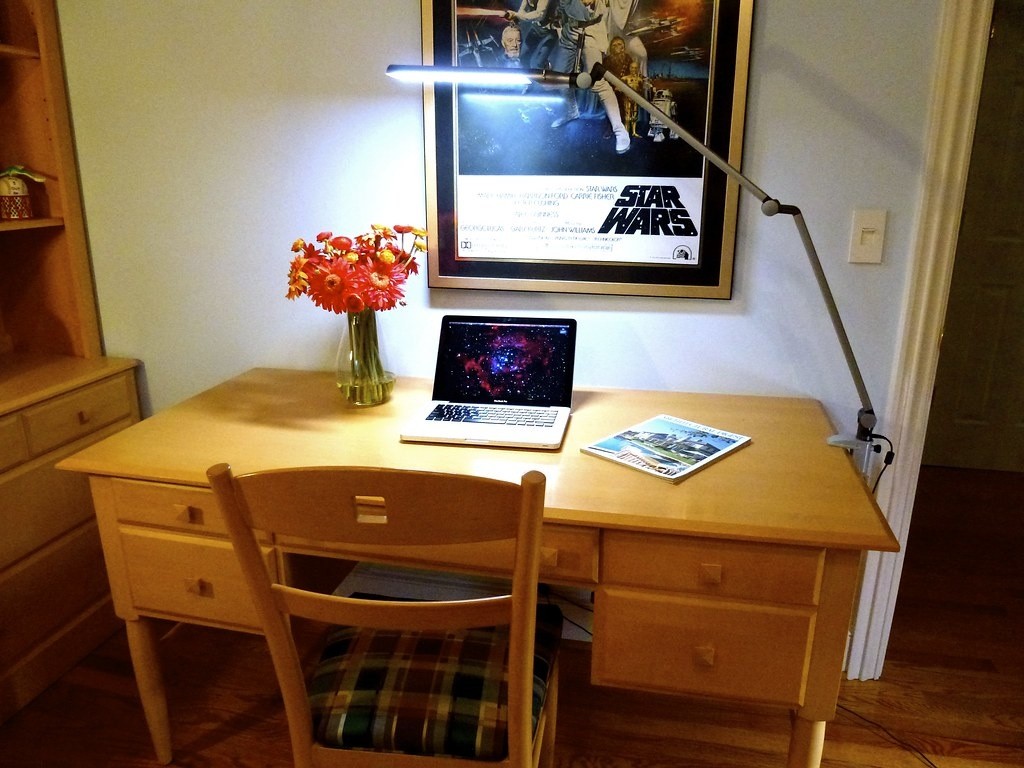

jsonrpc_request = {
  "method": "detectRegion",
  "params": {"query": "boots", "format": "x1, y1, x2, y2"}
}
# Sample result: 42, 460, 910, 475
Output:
551, 87, 580, 128
601, 92, 631, 155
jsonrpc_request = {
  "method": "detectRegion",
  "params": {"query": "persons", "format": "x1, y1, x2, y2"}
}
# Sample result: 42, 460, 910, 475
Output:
501, 0, 648, 154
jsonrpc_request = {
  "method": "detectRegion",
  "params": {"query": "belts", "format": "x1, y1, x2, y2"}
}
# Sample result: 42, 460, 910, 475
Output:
568, 14, 603, 28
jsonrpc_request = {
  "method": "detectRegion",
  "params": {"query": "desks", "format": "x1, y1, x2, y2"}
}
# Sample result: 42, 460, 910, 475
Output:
56, 367, 901, 768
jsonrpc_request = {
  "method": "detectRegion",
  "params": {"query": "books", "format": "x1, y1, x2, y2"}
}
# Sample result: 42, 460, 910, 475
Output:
580, 413, 752, 484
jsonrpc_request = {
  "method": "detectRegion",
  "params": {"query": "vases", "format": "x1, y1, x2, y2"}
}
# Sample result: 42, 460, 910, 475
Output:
335, 304, 397, 405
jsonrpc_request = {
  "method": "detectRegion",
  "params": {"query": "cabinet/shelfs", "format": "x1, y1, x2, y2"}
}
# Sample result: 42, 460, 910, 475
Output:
0, 0, 143, 731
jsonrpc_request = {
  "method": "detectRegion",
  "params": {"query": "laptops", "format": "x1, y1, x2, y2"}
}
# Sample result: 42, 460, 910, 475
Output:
400, 315, 577, 449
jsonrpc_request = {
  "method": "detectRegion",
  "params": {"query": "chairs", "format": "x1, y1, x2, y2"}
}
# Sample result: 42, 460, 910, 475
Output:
206, 462, 565, 768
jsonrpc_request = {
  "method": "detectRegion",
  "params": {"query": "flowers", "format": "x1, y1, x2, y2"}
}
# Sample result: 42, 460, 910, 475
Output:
285, 225, 428, 403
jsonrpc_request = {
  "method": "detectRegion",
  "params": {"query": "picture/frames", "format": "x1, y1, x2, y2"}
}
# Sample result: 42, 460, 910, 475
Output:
419, 0, 758, 301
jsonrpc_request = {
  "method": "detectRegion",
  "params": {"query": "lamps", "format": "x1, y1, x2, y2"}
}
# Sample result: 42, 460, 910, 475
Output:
382, 64, 883, 457
